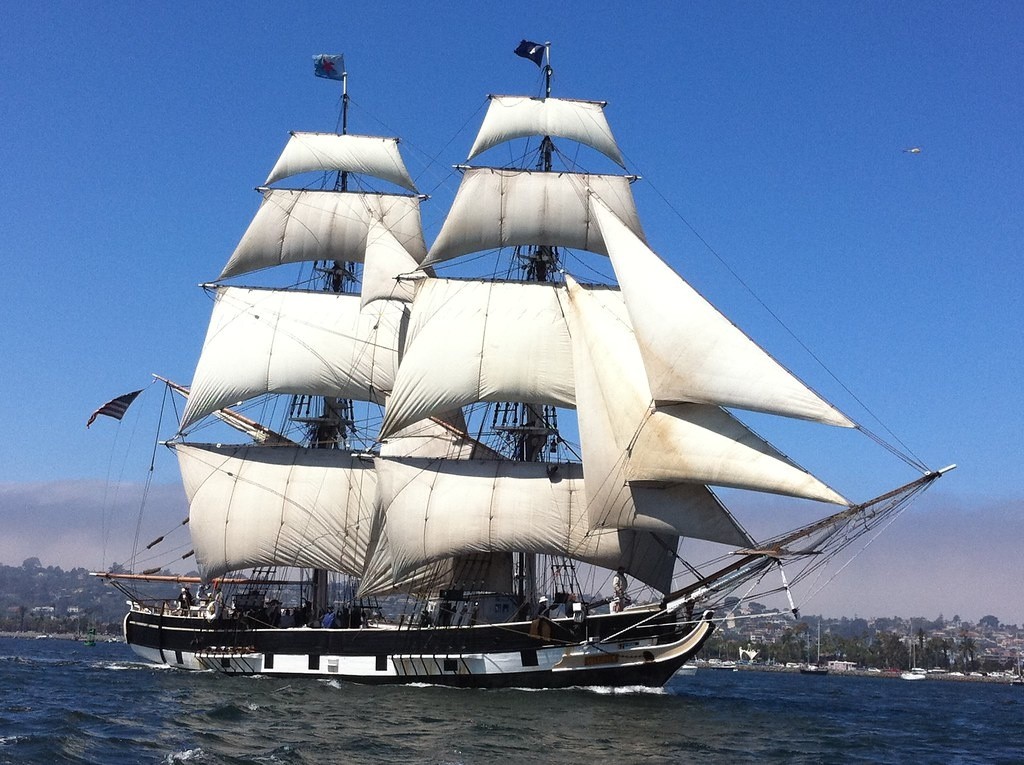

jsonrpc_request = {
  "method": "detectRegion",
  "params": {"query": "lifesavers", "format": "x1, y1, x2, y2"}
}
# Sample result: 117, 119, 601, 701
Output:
205, 601, 221, 620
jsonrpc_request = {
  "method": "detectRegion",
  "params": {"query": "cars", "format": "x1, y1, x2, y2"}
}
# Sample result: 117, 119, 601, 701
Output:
708, 658, 799, 668
968, 671, 983, 678
867, 666, 882, 674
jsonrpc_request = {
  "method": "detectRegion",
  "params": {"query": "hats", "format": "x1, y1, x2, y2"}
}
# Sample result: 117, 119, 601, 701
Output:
327, 605, 334, 609
539, 596, 548, 604
618, 567, 626, 570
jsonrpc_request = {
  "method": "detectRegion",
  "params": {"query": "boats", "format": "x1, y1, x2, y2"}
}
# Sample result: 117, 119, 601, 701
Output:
800, 661, 828, 676
681, 662, 699, 671
900, 669, 926, 680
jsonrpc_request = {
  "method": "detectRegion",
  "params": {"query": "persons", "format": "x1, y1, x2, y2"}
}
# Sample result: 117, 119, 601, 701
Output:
337, 607, 352, 628
428, 610, 435, 626
538, 595, 548, 617
398, 614, 405, 630
178, 583, 225, 619
565, 592, 578, 617
612, 566, 628, 613
269, 607, 296, 629
407, 610, 416, 630
469, 600, 480, 625
456, 602, 468, 626
321, 604, 335, 628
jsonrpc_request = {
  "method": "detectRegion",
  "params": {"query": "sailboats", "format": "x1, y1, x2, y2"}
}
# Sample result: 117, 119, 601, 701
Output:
86, 39, 1024, 692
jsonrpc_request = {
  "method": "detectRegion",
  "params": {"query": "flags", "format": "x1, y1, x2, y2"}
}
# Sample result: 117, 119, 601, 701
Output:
86, 387, 146, 429
